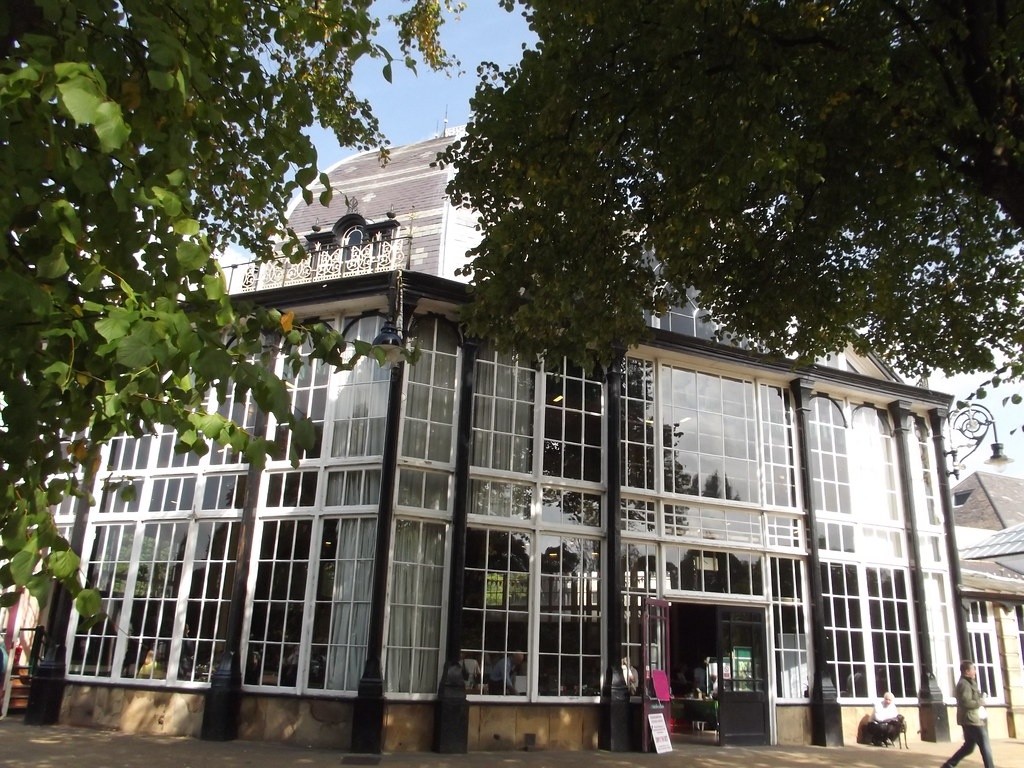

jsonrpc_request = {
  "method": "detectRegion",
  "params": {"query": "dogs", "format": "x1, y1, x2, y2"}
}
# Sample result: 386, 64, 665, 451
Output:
862, 714, 909, 749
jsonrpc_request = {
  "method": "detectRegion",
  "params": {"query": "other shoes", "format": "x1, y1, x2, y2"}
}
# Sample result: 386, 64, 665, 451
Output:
872, 738, 882, 746
882, 740, 892, 745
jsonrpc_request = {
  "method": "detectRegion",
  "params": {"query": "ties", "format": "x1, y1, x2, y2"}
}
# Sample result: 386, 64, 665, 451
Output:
461, 659, 469, 681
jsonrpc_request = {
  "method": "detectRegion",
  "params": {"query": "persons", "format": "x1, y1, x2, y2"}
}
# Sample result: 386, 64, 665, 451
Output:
459, 645, 523, 695
940, 661, 995, 768
179, 623, 196, 680
871, 692, 898, 746
622, 657, 638, 695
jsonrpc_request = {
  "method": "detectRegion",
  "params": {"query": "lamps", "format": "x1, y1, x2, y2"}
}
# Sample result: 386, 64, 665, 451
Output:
372, 267, 405, 350
943, 403, 1015, 481
552, 392, 564, 403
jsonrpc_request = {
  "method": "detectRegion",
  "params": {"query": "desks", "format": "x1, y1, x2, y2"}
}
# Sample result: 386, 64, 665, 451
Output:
670, 698, 718, 742
